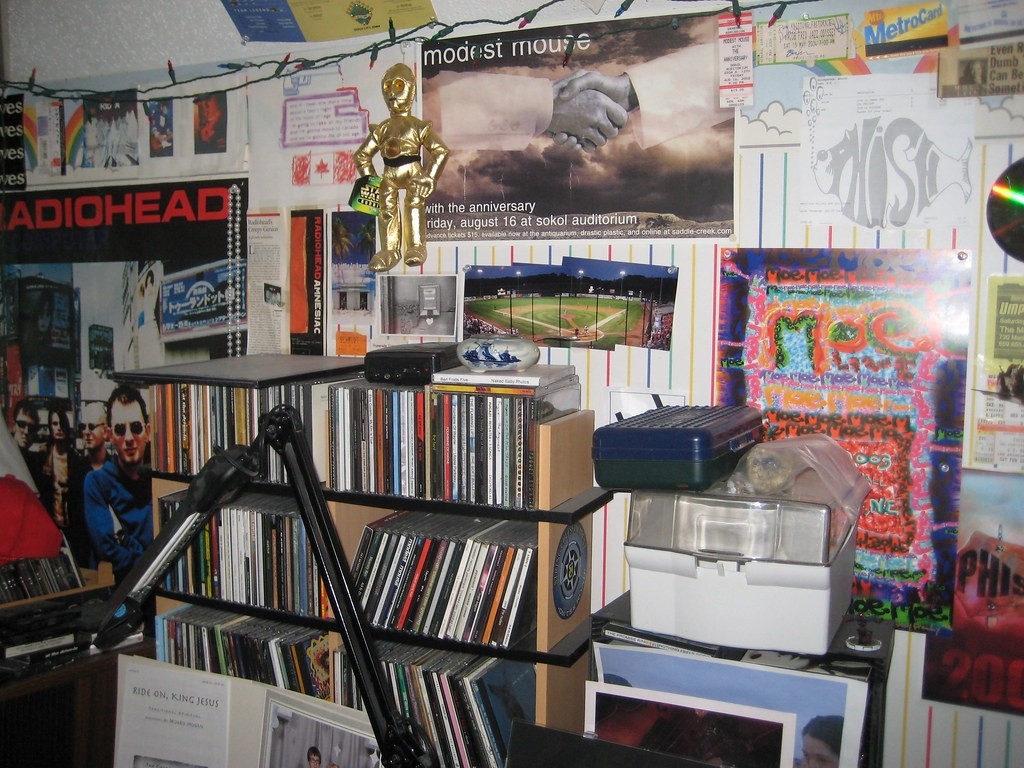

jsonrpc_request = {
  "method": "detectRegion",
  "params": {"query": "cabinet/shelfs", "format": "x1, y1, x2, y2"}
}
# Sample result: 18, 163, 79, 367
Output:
142, 387, 614, 735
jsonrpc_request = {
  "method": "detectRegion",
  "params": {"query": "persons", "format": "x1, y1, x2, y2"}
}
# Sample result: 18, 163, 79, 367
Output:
553, 40, 734, 155
353, 64, 450, 272
307, 746, 321, 768
422, 68, 629, 150
11, 386, 156, 632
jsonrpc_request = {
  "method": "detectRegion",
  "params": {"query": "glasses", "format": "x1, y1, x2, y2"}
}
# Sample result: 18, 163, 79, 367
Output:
79, 423, 105, 431
14, 420, 33, 430
113, 421, 146, 436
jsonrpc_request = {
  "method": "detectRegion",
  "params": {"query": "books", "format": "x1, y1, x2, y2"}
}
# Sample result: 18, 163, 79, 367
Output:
1, 536, 145, 666
111, 652, 386, 767
147, 361, 580, 513
157, 488, 540, 651
151, 603, 542, 768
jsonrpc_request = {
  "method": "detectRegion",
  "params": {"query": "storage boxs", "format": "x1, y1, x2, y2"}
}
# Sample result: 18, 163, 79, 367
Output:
625, 489, 858, 658
591, 405, 762, 493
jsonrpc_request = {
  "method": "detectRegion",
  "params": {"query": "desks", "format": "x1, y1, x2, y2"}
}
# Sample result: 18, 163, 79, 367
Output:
0, 643, 145, 768
